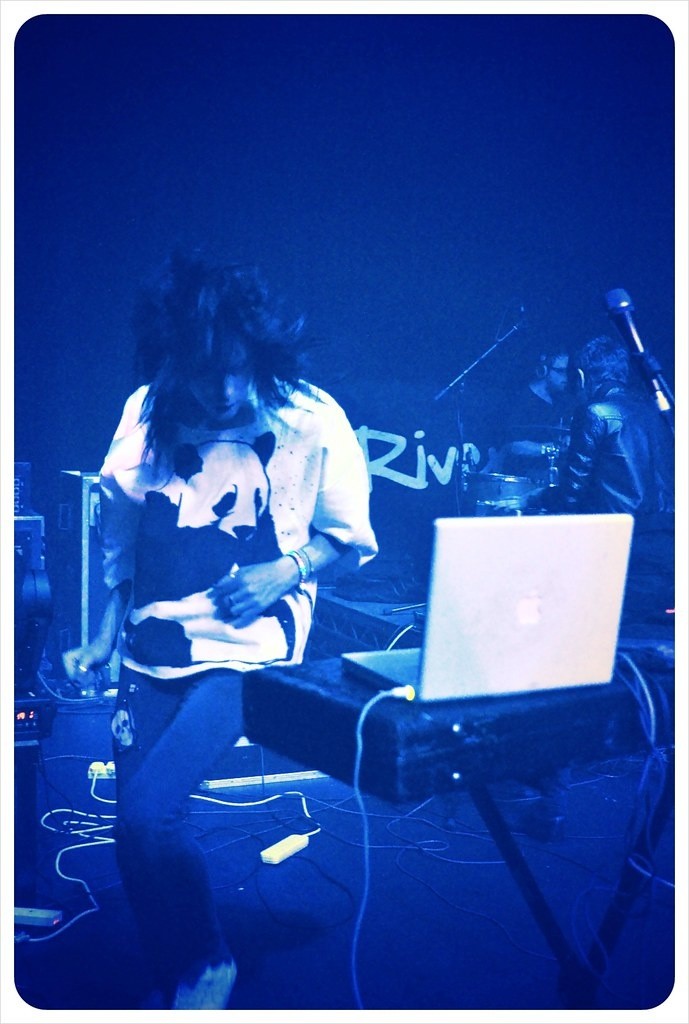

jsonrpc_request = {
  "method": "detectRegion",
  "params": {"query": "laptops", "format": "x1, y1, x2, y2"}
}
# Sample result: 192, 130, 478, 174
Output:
341, 507, 636, 708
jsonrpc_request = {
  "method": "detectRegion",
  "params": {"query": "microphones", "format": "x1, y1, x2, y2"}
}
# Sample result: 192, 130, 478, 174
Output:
602, 287, 671, 416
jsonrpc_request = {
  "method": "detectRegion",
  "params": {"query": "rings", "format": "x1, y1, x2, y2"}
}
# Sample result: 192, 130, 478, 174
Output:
228, 594, 236, 606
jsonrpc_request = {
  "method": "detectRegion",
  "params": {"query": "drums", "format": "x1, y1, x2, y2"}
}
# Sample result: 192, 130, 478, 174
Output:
461, 470, 545, 517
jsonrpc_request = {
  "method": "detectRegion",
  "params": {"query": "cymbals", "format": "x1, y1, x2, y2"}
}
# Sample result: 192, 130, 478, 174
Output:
510, 421, 572, 434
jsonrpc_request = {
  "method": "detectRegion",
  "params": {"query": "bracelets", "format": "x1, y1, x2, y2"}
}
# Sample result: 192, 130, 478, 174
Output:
286, 548, 314, 590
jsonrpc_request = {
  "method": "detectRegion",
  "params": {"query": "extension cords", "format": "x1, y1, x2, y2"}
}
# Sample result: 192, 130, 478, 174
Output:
89, 759, 121, 779
14, 906, 63, 928
258, 832, 311, 866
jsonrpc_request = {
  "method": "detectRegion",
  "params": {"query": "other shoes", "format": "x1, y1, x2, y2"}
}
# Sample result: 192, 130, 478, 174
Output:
174, 953, 237, 1010
145, 990, 168, 1008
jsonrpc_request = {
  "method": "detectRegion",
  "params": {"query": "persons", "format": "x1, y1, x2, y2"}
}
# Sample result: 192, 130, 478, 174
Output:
61, 241, 379, 1009
500, 325, 675, 841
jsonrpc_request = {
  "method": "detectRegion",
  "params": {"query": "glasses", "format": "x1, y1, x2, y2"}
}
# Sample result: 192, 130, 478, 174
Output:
551, 367, 565, 375
